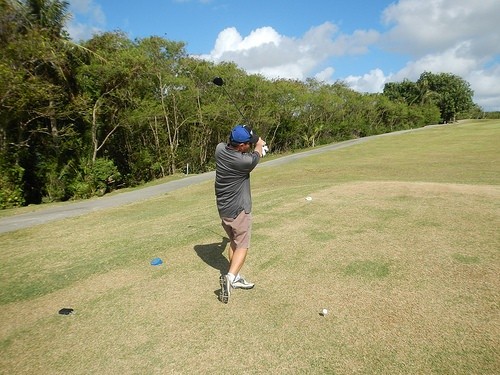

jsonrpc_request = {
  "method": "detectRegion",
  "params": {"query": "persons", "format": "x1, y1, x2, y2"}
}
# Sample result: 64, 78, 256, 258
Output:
215, 123, 266, 304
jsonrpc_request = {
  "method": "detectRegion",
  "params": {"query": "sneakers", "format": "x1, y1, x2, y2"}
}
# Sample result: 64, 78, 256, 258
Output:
231, 278, 255, 290
219, 274, 231, 304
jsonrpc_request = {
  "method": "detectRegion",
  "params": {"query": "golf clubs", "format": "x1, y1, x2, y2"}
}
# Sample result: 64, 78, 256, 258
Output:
212, 77, 269, 153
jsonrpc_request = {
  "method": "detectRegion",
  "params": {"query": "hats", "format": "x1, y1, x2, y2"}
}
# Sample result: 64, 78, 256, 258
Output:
231, 123, 259, 143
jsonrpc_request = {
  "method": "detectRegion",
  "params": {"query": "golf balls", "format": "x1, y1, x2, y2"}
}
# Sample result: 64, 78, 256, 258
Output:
322, 309, 328, 314
